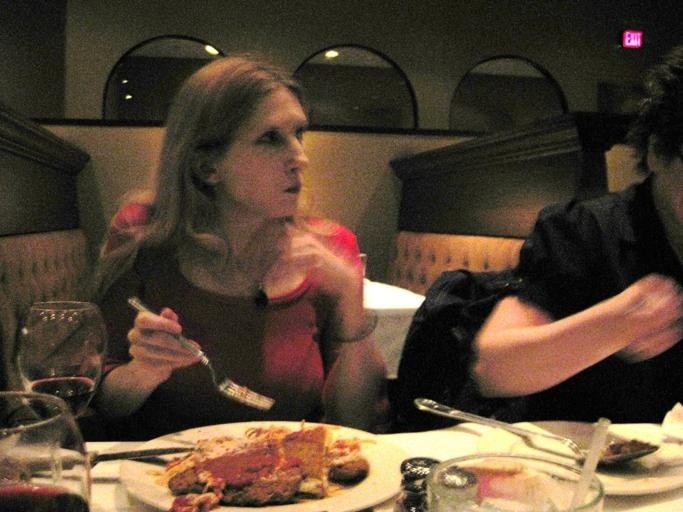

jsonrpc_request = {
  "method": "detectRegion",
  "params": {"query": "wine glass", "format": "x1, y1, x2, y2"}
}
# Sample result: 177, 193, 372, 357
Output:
17, 299, 108, 488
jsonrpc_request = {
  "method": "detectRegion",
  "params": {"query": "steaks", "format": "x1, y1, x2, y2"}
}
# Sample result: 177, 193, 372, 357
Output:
168, 426, 329, 506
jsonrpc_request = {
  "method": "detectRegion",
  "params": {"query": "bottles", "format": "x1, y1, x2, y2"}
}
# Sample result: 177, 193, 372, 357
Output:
395, 458, 440, 512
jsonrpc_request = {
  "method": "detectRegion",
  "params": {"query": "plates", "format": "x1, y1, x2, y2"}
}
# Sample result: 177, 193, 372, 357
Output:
120, 420, 405, 512
475, 415, 683, 500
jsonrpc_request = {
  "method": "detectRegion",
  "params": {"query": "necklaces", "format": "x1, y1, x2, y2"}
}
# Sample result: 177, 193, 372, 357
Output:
236, 252, 287, 309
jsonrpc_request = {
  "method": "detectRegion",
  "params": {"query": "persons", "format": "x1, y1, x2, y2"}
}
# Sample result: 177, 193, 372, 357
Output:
85, 56, 385, 440
468, 44, 683, 424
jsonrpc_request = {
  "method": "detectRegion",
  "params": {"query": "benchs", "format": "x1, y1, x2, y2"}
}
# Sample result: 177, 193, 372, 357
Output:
0, 108, 106, 410
385, 127, 587, 380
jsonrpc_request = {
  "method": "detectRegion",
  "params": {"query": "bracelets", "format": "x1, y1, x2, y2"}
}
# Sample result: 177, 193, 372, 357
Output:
332, 311, 378, 342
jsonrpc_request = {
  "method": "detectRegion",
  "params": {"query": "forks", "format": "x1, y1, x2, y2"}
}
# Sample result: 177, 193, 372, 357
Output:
129, 294, 277, 413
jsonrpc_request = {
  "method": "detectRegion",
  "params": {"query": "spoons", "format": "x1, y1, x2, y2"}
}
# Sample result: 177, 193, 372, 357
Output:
410, 392, 658, 469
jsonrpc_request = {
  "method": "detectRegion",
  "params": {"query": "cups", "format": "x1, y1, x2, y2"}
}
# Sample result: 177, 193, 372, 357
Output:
0, 391, 90, 510
425, 452, 605, 512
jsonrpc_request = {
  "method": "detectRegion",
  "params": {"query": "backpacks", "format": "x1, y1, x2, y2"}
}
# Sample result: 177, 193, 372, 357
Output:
393, 268, 513, 430
